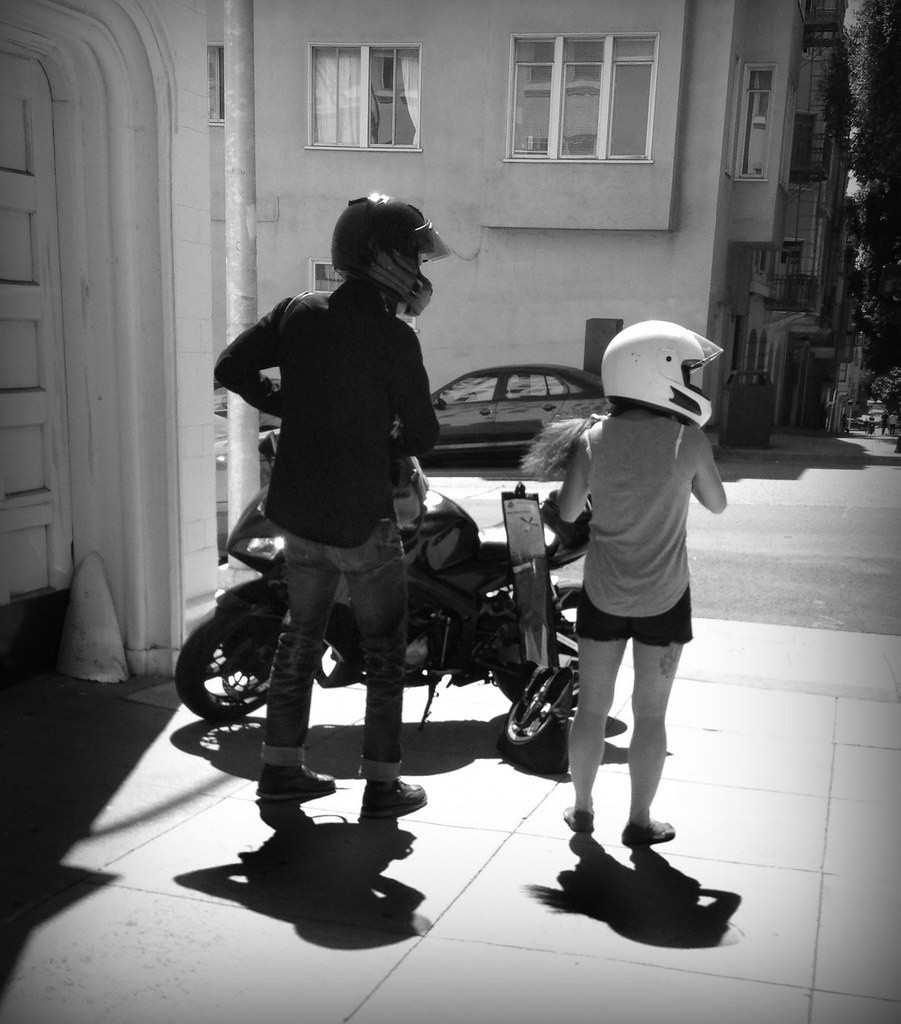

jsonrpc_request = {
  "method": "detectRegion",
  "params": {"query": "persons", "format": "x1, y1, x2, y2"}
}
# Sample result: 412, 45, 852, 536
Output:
880, 411, 898, 435
519, 320, 727, 846
215, 195, 450, 819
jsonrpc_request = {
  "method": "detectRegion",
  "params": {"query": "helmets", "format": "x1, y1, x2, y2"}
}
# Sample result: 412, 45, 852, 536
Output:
602, 320, 712, 428
332, 195, 432, 316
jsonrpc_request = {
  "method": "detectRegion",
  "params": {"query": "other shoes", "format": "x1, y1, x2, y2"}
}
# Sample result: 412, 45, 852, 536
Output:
257, 764, 335, 800
360, 779, 427, 817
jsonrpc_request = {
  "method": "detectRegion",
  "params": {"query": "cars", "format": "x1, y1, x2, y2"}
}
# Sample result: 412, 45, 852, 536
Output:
214, 411, 287, 566
405, 362, 613, 470
847, 418, 865, 431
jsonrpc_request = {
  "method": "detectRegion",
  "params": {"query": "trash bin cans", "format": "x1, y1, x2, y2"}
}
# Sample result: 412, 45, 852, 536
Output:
718, 369, 778, 449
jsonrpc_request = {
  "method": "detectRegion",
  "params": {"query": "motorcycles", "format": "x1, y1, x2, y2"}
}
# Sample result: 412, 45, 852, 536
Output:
175, 426, 609, 744
868, 421, 876, 434
